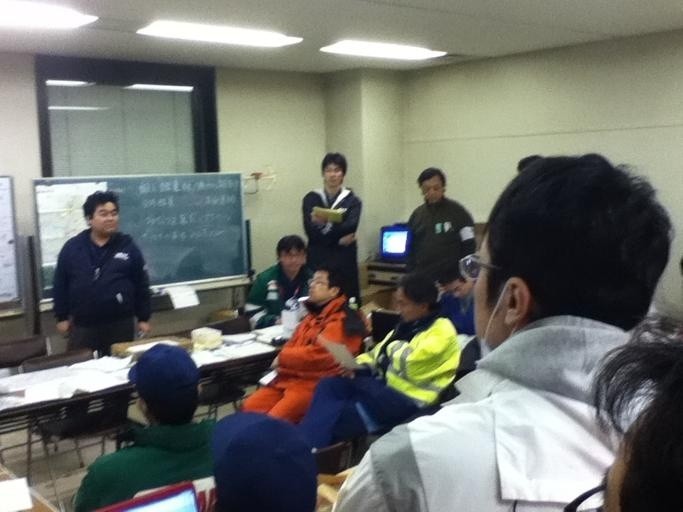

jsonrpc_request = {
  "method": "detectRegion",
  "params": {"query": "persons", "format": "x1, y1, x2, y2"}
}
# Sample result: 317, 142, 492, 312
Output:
238, 264, 366, 425
70, 342, 219, 510
589, 312, 683, 511
331, 155, 675, 512
433, 258, 478, 339
301, 153, 362, 306
404, 167, 479, 285
51, 188, 151, 438
295, 272, 461, 452
242, 232, 316, 329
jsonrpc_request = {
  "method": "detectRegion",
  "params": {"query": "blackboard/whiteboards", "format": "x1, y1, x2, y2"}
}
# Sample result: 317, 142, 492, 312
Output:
32, 172, 251, 312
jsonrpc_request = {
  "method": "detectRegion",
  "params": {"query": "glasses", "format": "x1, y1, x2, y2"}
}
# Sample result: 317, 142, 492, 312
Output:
458, 251, 498, 283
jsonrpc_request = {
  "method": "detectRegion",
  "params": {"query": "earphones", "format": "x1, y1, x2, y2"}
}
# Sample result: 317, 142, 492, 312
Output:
479, 338, 491, 357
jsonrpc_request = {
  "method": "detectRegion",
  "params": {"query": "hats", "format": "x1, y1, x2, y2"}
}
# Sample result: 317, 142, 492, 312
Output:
128, 342, 201, 413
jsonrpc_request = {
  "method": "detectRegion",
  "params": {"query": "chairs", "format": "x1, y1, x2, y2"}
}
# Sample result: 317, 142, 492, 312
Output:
0, 259, 481, 511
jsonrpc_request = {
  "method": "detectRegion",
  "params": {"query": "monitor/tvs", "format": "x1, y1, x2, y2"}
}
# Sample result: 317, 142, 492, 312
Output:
381, 225, 411, 261
